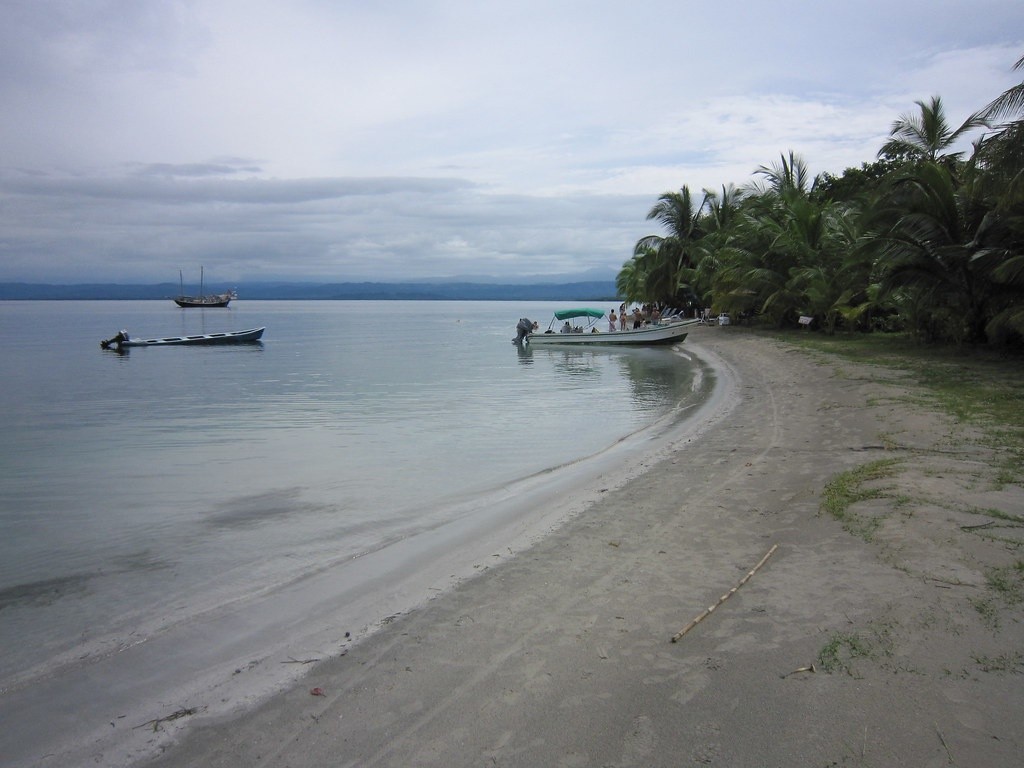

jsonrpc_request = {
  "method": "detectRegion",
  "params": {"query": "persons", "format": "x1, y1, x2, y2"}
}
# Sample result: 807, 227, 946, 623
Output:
619, 304, 625, 315
529, 322, 539, 333
650, 308, 661, 325
609, 309, 617, 332
620, 313, 626, 331
632, 307, 646, 330
560, 322, 571, 334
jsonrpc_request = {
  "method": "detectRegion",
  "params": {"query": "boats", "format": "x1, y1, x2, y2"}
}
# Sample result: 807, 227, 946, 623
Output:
168, 265, 237, 308
524, 308, 702, 347
116, 326, 265, 347
